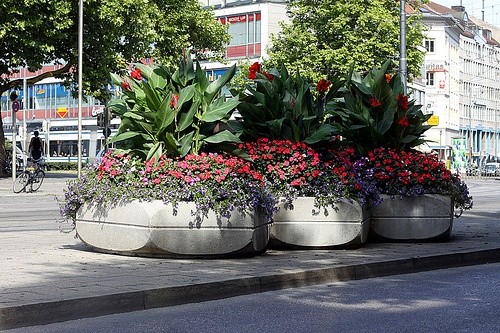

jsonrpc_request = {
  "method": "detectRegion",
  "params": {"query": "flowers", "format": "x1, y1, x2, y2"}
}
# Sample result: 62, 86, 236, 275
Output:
52, 58, 476, 240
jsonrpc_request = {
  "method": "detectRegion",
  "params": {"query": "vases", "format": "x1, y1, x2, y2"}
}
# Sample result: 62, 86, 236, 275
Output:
367, 192, 457, 242
268, 194, 366, 248
72, 192, 270, 259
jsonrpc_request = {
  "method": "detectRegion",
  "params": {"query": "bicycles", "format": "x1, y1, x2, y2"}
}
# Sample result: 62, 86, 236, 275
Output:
12, 158, 45, 194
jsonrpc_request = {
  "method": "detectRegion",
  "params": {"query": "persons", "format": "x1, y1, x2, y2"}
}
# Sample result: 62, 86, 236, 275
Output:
28, 131, 44, 166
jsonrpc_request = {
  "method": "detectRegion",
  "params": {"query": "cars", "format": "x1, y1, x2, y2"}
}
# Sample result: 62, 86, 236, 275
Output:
466, 163, 500, 176
